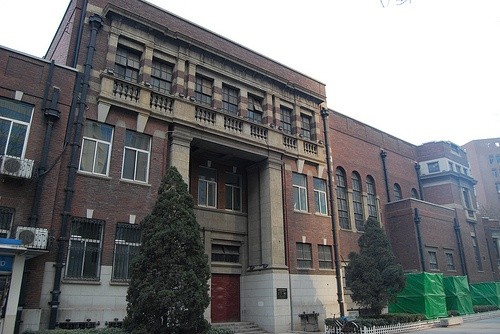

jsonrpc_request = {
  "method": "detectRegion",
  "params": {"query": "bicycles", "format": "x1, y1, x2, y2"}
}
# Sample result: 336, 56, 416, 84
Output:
325, 311, 359, 334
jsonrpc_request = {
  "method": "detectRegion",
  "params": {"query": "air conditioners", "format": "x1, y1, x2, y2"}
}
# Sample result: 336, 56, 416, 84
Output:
0, 154, 35, 180
16, 225, 49, 250
